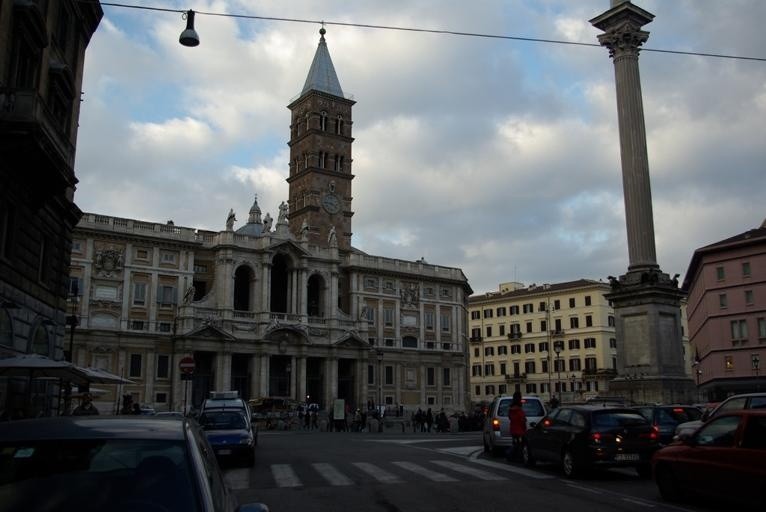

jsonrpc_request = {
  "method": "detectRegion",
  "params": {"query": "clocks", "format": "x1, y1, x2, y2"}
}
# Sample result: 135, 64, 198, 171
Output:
322, 194, 341, 215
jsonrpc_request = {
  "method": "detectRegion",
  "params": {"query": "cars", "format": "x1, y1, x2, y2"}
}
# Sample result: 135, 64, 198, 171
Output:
0, 411, 271, 511
128, 390, 259, 468
481, 391, 765, 509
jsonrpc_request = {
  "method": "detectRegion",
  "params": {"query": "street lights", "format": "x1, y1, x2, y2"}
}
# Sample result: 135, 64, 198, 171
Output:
571, 373, 576, 392
554, 340, 563, 403
376, 350, 385, 417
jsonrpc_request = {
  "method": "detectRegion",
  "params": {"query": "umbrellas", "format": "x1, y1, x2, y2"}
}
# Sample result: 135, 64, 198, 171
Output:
82, 363, 136, 392
55, 356, 114, 416
0, 351, 72, 408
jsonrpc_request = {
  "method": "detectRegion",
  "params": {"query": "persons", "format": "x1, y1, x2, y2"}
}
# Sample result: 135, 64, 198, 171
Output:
264, 399, 469, 433
506, 390, 530, 464
549, 395, 560, 409
119, 400, 132, 415
130, 402, 143, 414
72, 393, 98, 415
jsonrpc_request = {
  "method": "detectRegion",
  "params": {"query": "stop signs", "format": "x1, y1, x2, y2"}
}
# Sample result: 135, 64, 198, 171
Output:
178, 357, 195, 374
